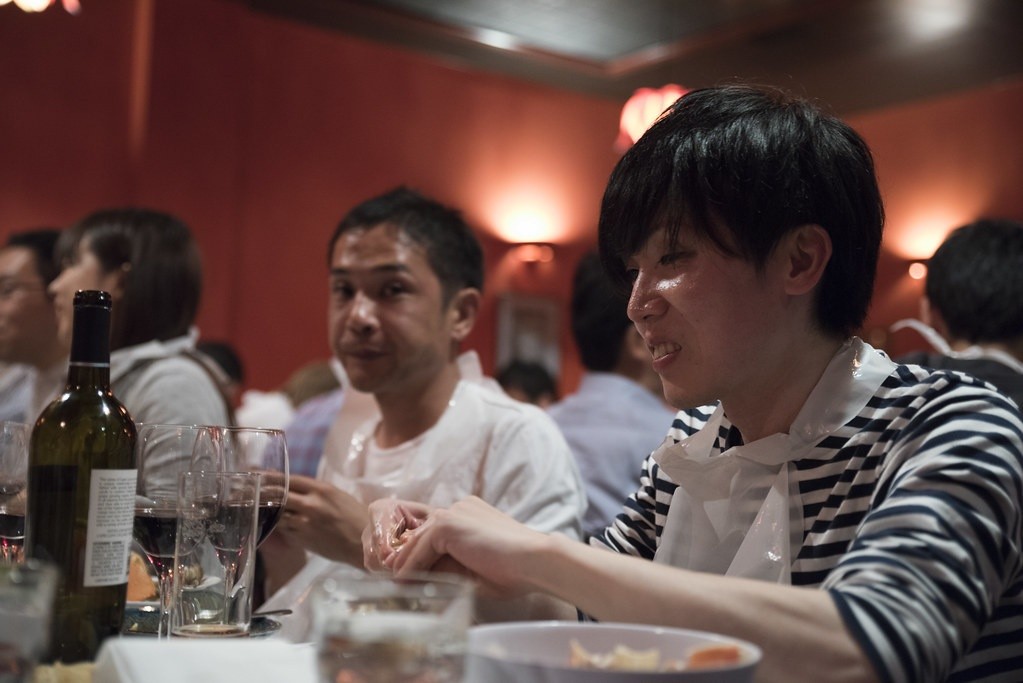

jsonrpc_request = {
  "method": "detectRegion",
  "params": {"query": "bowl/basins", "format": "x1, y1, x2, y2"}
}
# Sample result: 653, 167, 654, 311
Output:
465, 621, 762, 683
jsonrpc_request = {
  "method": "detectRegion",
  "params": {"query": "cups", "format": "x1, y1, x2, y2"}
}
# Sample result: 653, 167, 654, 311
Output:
168, 471, 263, 642
0, 562, 59, 683
312, 572, 477, 682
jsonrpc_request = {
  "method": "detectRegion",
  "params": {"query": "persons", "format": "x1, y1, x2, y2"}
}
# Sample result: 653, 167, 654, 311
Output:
0, 82, 1023, 683
233, 184, 588, 627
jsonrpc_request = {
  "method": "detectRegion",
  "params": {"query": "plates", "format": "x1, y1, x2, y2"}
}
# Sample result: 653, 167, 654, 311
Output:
124, 603, 280, 637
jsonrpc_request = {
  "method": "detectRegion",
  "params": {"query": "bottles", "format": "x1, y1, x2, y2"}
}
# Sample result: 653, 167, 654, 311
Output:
25, 288, 139, 665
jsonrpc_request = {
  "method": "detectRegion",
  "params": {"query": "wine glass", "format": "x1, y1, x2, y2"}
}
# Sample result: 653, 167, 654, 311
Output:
134, 421, 227, 639
1, 419, 34, 564
188, 424, 289, 633
